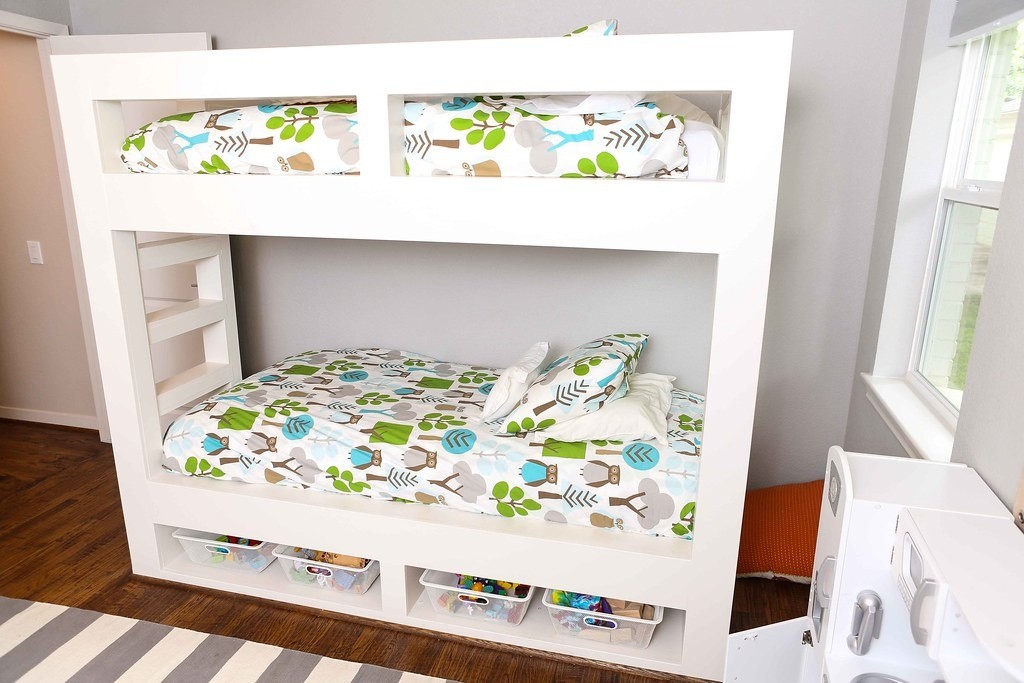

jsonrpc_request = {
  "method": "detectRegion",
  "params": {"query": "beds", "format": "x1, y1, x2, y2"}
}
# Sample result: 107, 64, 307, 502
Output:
44, 29, 795, 683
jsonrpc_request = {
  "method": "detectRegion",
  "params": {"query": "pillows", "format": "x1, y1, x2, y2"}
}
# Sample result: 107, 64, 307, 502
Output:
494, 332, 649, 436
521, 92, 648, 115
481, 341, 552, 423
481, 19, 619, 102
533, 373, 676, 447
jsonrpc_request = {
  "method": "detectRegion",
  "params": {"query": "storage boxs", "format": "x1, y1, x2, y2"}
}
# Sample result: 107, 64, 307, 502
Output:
272, 544, 379, 595
172, 528, 276, 573
542, 589, 664, 649
418, 570, 535, 626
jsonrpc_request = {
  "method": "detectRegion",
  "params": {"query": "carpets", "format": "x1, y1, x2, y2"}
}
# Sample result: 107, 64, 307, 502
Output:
0, 596, 456, 683
